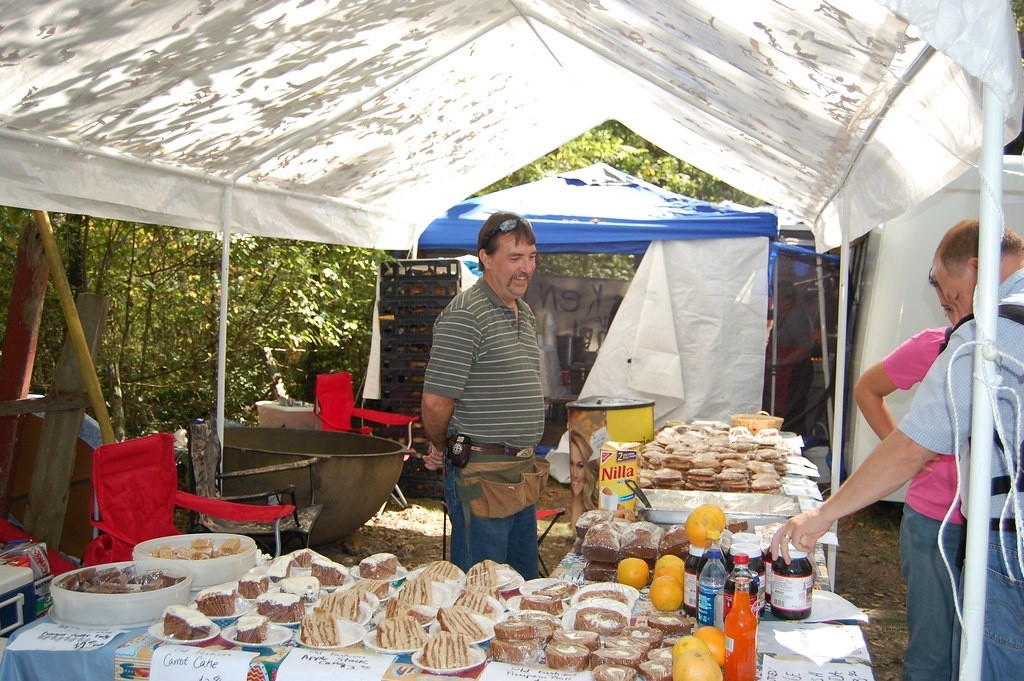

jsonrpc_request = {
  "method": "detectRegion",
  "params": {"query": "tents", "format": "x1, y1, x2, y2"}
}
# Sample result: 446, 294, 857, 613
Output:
393, 160, 855, 416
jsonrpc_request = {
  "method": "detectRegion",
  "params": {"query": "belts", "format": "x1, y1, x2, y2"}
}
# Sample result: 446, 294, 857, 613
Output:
989, 517, 1017, 531
448, 439, 538, 463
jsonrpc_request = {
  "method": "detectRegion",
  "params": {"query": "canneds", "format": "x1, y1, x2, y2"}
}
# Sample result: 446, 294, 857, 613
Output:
682, 529, 814, 621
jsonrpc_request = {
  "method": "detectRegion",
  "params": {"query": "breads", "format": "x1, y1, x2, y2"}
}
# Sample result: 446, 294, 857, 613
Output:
56, 566, 182, 594
638, 428, 787, 496
490, 583, 692, 681
151, 537, 247, 560
574, 509, 688, 581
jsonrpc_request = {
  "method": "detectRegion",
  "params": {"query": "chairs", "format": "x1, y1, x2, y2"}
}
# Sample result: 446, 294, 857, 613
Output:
312, 370, 421, 526
440, 500, 566, 578
185, 418, 325, 554
82, 432, 296, 568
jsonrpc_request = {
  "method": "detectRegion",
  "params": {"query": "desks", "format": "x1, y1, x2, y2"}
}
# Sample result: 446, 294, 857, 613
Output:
549, 431, 832, 592
0, 586, 875, 681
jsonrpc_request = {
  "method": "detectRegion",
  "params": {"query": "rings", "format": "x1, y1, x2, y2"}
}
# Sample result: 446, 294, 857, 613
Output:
783, 533, 791, 541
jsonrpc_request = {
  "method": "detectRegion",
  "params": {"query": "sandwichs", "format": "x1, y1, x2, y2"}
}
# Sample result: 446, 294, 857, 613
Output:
164, 552, 512, 669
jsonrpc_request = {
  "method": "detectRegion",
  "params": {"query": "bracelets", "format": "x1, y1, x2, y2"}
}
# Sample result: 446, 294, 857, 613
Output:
431, 444, 443, 457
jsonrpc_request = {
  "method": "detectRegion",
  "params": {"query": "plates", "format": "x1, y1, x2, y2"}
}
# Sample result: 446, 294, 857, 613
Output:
147, 565, 639, 675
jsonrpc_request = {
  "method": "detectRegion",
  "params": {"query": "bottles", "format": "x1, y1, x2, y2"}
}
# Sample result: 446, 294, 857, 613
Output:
721, 552, 758, 625
683, 543, 708, 617
725, 532, 766, 589
725, 543, 766, 617
764, 539, 796, 605
696, 537, 729, 625
770, 543, 814, 621
697, 549, 727, 629
723, 576, 758, 681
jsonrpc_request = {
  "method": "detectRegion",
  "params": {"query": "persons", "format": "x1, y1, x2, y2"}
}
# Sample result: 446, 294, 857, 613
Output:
422, 210, 550, 583
771, 217, 1024, 681
771, 283, 814, 437
570, 431, 599, 533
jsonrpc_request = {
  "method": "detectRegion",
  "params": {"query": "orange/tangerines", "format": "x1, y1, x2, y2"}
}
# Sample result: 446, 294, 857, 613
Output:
617, 555, 687, 611
672, 625, 728, 681
685, 504, 726, 547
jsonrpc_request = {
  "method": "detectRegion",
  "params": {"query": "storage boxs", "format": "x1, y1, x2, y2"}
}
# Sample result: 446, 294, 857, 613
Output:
598, 440, 643, 510
34, 573, 55, 616
0, 564, 37, 638
8, 540, 51, 581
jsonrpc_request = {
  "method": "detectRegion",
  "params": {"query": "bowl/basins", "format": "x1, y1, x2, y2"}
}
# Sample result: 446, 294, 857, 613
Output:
131, 532, 258, 588
49, 561, 193, 626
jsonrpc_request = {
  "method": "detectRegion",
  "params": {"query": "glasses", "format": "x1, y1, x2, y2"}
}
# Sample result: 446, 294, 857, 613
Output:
485, 217, 532, 251
